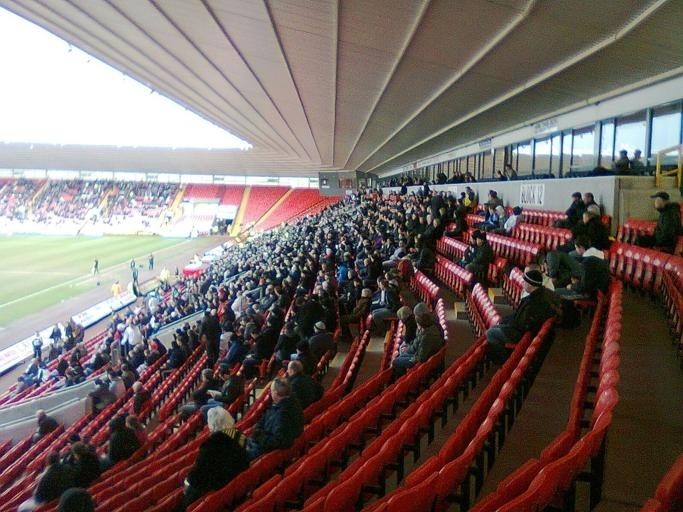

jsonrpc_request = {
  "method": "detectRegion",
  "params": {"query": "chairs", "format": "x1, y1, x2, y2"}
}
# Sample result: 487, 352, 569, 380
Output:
0, 205, 681, 512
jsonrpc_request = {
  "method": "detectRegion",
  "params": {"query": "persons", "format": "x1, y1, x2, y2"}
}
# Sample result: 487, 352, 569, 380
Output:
633, 191, 683, 248
613, 151, 628, 173
0, 165, 612, 512
629, 150, 643, 169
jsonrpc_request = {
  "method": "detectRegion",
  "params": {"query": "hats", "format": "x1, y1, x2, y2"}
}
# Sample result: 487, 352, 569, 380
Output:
650, 192, 669, 199
471, 230, 486, 241
523, 271, 542, 289
414, 303, 429, 317
314, 322, 326, 330
